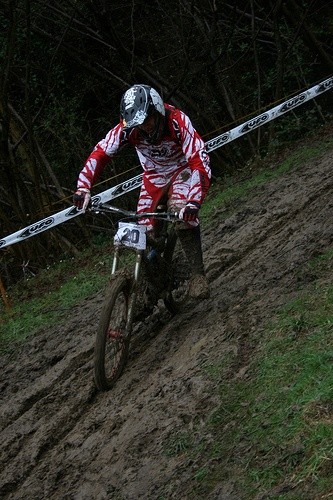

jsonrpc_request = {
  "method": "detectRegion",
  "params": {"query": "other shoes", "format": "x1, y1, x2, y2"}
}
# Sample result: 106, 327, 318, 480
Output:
187, 274, 210, 300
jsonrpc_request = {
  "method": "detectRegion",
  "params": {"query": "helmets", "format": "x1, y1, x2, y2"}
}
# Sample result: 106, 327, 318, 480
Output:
118, 83, 167, 142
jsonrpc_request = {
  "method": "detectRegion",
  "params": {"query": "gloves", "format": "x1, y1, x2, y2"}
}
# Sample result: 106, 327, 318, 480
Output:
71, 188, 92, 210
178, 200, 201, 221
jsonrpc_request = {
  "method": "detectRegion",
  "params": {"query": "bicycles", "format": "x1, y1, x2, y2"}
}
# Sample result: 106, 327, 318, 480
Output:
60, 185, 192, 391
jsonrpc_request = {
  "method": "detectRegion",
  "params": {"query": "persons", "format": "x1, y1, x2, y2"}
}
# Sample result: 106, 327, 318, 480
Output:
72, 84, 211, 299
